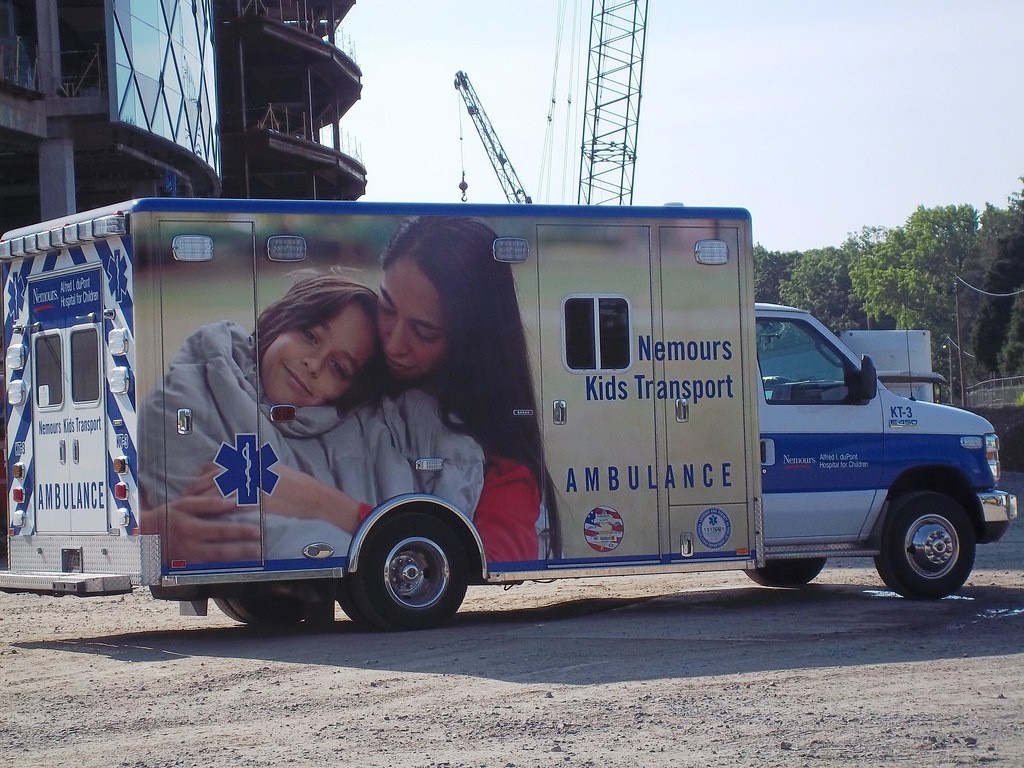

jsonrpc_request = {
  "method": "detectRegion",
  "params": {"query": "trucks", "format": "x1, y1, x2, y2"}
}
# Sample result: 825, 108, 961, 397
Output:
1, 199, 1017, 634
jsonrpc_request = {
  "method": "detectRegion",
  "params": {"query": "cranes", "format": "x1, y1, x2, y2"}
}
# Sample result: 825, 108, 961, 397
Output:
452, 70, 537, 205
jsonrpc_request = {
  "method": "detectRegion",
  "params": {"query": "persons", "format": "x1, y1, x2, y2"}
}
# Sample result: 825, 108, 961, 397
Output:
135, 218, 564, 566
